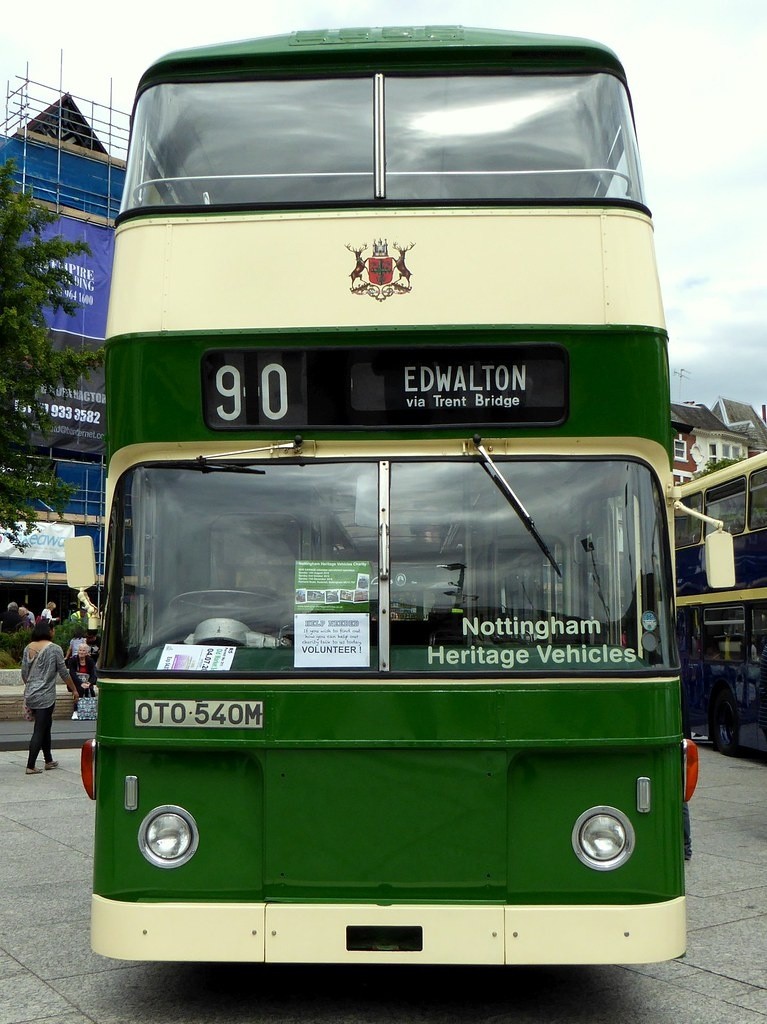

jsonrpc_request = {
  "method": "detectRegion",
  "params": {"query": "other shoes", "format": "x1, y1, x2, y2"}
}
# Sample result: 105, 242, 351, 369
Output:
44, 761, 58, 770
72, 709, 78, 721
25, 767, 43, 774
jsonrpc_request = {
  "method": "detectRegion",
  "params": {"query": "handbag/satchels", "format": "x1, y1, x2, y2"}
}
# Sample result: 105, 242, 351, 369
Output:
76, 688, 99, 721
22, 696, 34, 722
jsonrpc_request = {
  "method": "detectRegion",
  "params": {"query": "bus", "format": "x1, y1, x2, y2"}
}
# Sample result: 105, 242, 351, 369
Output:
61, 25, 734, 971
673, 451, 767, 761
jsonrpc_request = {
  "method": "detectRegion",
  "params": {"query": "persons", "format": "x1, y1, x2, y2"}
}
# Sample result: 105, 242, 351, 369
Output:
41, 600, 67, 624
85, 629, 101, 697
758, 644, 767, 740
67, 643, 96, 719
71, 605, 87, 628
2, 601, 36, 633
63, 626, 86, 657
730, 504, 745, 534
21, 618, 79, 773
739, 634, 766, 663
